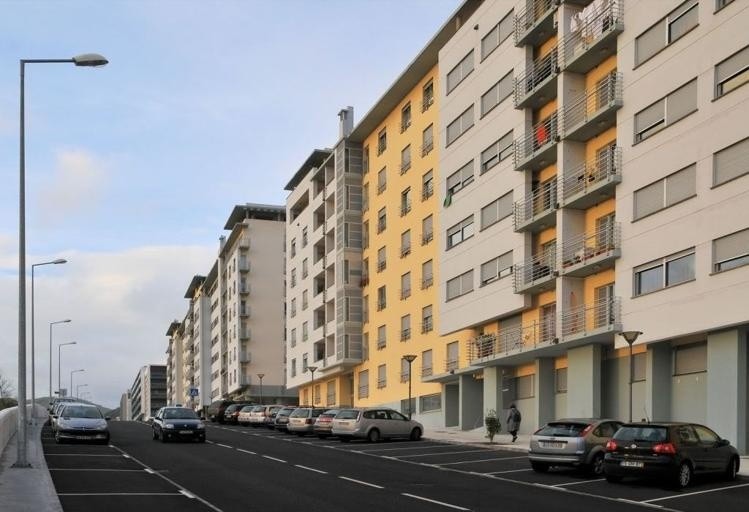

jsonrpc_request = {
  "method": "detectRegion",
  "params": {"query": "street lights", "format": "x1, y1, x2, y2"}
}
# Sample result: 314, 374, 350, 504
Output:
13, 52, 111, 468
618, 331, 645, 423
308, 366, 318, 405
256, 373, 265, 403
403, 355, 418, 419
28, 256, 91, 423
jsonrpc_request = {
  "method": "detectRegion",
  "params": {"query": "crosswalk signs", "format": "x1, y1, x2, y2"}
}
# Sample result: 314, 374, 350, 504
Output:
191, 389, 198, 396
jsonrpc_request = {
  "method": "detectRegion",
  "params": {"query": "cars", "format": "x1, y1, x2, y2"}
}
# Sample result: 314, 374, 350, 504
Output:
46, 397, 110, 445
151, 406, 206, 443
206, 398, 341, 440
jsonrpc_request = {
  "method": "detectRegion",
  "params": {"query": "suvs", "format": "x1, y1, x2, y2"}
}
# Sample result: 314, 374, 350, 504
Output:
528, 418, 626, 476
331, 408, 424, 442
604, 423, 741, 488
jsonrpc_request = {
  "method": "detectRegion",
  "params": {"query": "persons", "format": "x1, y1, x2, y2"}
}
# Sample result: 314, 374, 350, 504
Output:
506, 403, 521, 443
